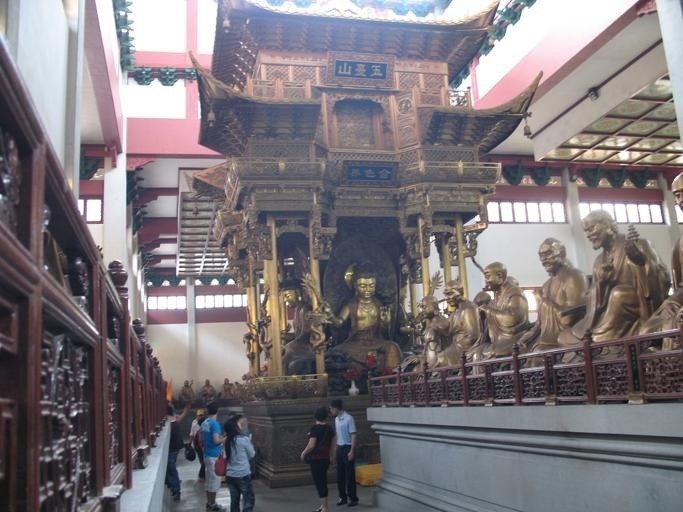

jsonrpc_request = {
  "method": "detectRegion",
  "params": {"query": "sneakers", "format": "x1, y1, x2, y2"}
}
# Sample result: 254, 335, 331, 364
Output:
206, 503, 226, 512
336, 498, 359, 507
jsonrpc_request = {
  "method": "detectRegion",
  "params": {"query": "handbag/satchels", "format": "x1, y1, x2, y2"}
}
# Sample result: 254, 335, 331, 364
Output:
184, 443, 196, 461
214, 454, 227, 475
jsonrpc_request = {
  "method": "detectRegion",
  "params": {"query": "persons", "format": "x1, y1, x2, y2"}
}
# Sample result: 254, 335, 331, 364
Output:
179, 380, 195, 401
328, 399, 359, 506
199, 379, 216, 405
300, 407, 334, 512
637, 172, 681, 352
275, 282, 314, 374
556, 209, 671, 362
219, 378, 235, 400
412, 237, 590, 379
324, 270, 404, 370
164, 401, 255, 512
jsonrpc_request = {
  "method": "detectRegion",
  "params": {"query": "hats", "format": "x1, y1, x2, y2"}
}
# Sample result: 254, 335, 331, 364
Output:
196, 408, 205, 416
